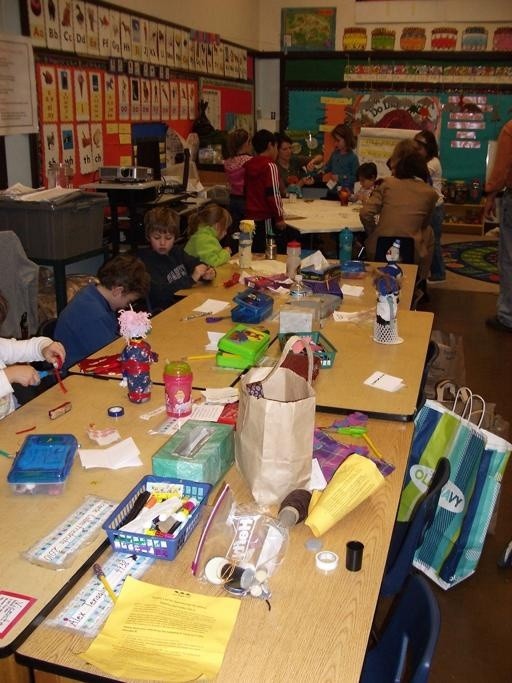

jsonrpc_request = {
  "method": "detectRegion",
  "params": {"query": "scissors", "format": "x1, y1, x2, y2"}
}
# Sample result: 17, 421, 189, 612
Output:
319, 426, 368, 435
206, 315, 231, 323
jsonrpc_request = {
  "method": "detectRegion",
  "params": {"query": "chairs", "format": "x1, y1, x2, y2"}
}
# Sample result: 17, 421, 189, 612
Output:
359, 574, 441, 683
374, 235, 415, 263
370, 456, 451, 644
411, 287, 424, 309
37, 318, 58, 388
416, 339, 440, 412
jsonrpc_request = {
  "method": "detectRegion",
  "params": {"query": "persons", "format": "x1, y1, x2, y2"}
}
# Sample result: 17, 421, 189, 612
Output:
483, 116, 512, 339
359, 138, 440, 308
354, 162, 378, 204
240, 128, 288, 254
53, 252, 150, 388
326, 123, 363, 258
139, 206, 217, 317
413, 131, 447, 282
185, 203, 233, 268
274, 133, 324, 197
224, 129, 255, 253
1, 293, 67, 421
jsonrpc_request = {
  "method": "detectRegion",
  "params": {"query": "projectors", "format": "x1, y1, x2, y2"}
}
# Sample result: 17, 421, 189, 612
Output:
99, 165, 155, 182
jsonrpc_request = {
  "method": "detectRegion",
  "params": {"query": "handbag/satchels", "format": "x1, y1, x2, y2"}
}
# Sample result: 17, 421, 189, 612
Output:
439, 381, 494, 431
391, 385, 512, 590
234, 336, 317, 508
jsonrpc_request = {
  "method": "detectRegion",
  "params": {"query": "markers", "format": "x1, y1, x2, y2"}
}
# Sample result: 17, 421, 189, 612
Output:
136, 495, 156, 518
118, 491, 149, 530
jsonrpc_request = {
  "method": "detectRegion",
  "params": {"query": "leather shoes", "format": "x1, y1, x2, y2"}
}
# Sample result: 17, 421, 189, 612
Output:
416, 291, 434, 308
484, 317, 512, 333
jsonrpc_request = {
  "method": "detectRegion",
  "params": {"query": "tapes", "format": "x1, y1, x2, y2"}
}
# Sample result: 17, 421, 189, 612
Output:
52, 357, 62, 370
316, 550, 339, 571
108, 406, 124, 416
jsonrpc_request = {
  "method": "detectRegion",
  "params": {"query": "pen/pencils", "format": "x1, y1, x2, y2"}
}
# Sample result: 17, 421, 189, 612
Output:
180, 312, 212, 321
182, 353, 215, 360
362, 434, 385, 463
0, 450, 14, 458
93, 564, 117, 604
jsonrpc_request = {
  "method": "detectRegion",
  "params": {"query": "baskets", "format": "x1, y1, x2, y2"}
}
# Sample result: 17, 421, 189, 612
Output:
278, 330, 337, 369
102, 474, 213, 561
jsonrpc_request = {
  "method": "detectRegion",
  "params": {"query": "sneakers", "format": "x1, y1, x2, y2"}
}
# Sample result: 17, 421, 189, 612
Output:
427, 276, 446, 283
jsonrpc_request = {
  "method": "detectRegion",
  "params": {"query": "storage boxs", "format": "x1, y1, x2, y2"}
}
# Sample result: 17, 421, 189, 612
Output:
0, 189, 109, 259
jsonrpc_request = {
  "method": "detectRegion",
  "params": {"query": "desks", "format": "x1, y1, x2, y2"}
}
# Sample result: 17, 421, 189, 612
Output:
0, 372, 228, 658
173, 251, 418, 310
14, 413, 414, 683
68, 293, 289, 391
232, 304, 434, 422
27, 245, 110, 318
280, 197, 380, 250
112, 191, 191, 254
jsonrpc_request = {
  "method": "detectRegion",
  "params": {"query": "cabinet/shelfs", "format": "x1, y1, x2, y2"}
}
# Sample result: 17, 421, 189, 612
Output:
440, 196, 486, 236
